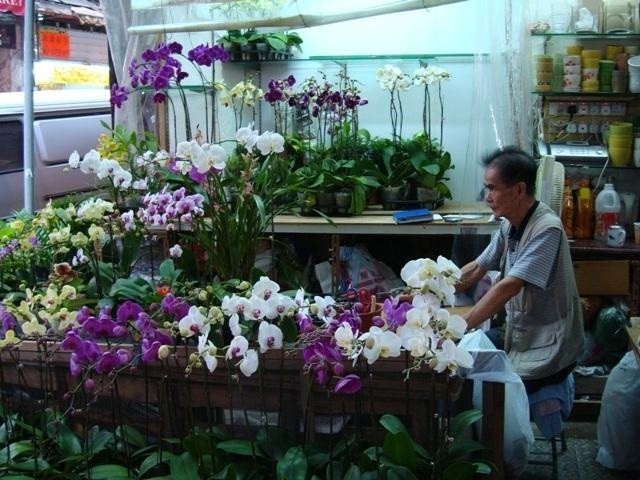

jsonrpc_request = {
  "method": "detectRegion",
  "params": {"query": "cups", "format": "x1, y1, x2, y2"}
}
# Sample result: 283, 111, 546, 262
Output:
607, 225, 627, 248
633, 222, 640, 245
575, 20, 592, 31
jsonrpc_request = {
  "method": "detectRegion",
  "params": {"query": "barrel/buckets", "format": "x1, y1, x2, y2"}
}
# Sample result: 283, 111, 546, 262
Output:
564, 177, 574, 240
594, 184, 621, 244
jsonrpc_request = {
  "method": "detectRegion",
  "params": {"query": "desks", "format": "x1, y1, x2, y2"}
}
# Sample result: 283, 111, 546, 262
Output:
130, 203, 493, 271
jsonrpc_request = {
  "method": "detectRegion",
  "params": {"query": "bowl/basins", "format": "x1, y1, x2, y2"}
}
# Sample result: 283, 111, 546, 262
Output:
633, 137, 640, 168
533, 45, 640, 95
608, 122, 633, 167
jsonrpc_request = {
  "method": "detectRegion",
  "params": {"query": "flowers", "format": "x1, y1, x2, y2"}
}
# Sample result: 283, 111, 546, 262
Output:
55, 2, 452, 221
2, 259, 497, 477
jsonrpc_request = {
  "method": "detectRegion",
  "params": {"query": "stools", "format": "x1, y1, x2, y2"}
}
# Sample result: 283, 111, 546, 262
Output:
527, 372, 568, 480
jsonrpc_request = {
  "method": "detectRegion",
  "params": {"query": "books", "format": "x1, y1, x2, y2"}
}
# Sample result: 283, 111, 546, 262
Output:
394, 207, 433, 225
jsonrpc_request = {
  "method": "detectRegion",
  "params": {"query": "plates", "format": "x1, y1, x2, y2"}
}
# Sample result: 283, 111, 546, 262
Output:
574, 28, 598, 34
606, 29, 631, 34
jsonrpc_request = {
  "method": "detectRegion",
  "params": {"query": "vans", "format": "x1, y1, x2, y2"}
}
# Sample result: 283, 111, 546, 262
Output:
0, 86, 113, 219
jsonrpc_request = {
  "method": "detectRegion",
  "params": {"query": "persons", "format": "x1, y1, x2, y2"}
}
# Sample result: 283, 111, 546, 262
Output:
403, 145, 585, 394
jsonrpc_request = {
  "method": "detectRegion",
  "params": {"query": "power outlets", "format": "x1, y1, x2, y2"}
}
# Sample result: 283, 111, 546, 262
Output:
549, 102, 626, 116
549, 119, 611, 134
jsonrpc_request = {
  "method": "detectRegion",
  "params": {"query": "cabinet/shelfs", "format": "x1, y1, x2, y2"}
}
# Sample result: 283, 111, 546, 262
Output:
530, 30, 640, 367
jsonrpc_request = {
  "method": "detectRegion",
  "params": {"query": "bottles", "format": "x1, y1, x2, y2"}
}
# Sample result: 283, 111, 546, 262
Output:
562, 180, 574, 238
